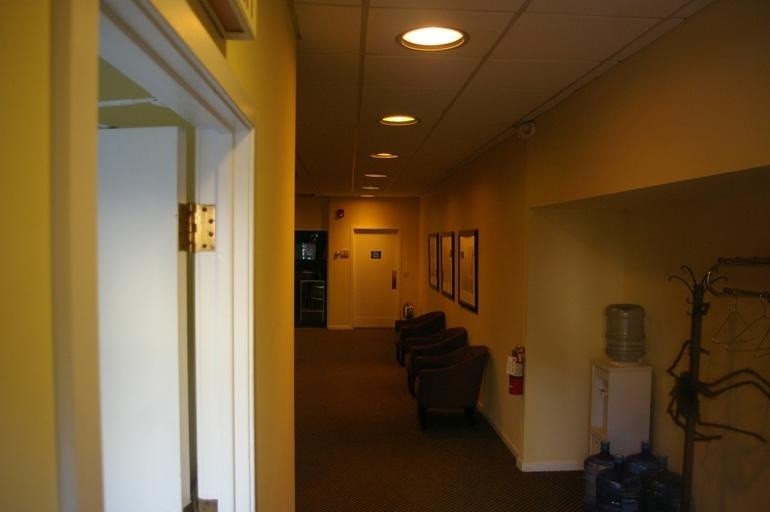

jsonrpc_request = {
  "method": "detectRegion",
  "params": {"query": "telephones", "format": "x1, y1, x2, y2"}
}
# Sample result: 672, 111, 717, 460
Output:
340, 248, 349, 258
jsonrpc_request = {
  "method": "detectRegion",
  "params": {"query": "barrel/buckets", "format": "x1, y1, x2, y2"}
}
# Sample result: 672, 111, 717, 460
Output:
596, 454, 644, 512
643, 451, 680, 511
624, 438, 660, 474
605, 301, 647, 362
582, 441, 622, 506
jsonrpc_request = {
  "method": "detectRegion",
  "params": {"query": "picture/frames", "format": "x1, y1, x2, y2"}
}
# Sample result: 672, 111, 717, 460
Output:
427, 229, 478, 313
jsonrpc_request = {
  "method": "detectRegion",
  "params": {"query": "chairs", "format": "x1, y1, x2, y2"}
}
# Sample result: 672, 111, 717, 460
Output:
395, 310, 490, 433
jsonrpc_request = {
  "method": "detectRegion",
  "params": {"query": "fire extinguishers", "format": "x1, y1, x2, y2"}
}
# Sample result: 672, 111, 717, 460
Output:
509, 347, 524, 395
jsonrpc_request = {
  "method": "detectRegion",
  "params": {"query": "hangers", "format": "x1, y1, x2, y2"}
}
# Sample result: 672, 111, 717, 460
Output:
711, 288, 770, 359
